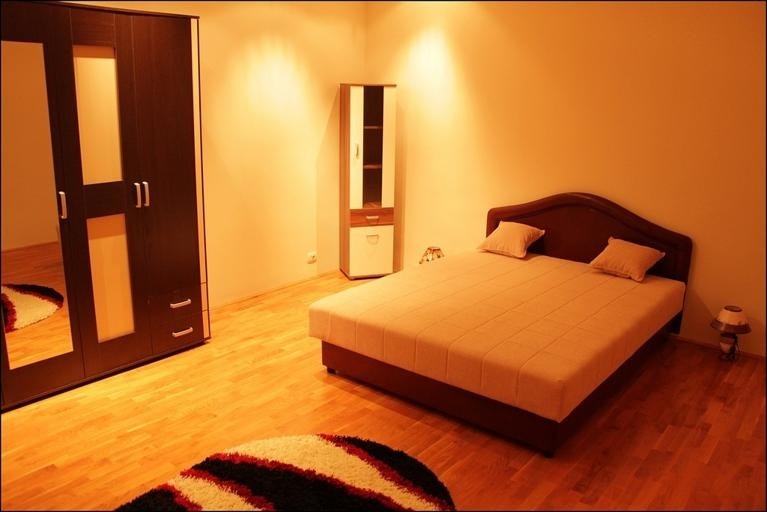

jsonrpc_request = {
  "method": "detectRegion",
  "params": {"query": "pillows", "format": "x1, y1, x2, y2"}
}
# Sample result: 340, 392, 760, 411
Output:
480, 220, 547, 259
590, 234, 668, 282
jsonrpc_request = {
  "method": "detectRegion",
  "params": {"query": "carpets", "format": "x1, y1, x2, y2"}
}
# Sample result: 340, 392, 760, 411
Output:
106, 431, 458, 510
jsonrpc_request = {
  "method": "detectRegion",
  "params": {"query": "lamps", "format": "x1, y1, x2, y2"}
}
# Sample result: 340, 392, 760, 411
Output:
418, 247, 445, 265
712, 305, 751, 362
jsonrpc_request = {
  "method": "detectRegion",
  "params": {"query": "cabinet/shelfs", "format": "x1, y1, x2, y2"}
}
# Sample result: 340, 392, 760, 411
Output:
339, 81, 398, 281
0, 0, 211, 410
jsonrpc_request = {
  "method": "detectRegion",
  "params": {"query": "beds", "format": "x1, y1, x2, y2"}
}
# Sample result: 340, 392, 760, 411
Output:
308, 192, 695, 459
